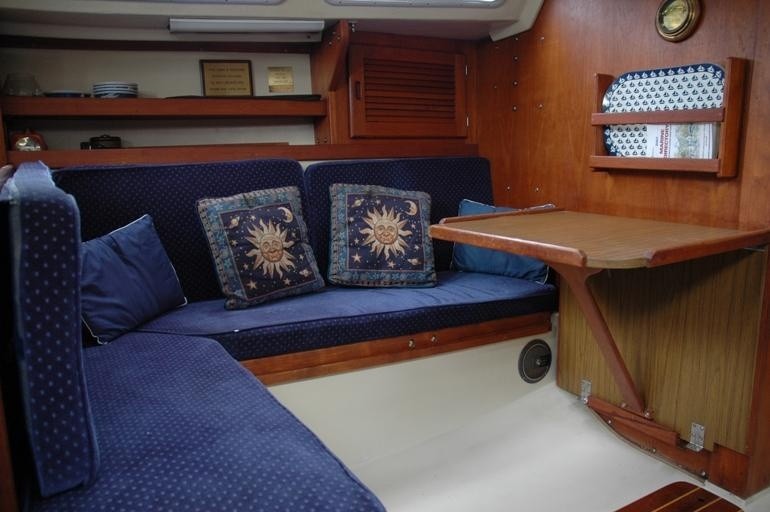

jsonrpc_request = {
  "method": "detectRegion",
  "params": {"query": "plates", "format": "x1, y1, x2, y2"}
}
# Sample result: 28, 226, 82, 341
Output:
92, 81, 137, 99
43, 90, 91, 98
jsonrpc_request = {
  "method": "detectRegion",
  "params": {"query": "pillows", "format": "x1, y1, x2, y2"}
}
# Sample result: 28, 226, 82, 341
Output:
195, 186, 323, 310
80, 213, 187, 342
328, 183, 436, 288
450, 199, 548, 283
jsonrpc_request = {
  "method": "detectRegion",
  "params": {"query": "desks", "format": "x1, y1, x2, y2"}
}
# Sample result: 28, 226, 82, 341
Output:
433, 197, 767, 415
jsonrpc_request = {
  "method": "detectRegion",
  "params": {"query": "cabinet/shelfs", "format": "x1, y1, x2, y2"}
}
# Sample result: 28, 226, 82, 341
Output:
332, 28, 479, 144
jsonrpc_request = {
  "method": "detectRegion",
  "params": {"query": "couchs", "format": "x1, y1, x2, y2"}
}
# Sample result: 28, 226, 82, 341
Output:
4, 147, 566, 511
